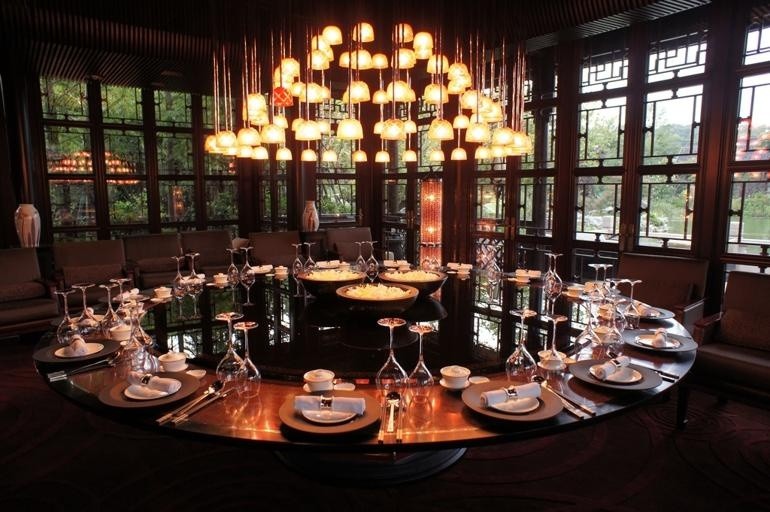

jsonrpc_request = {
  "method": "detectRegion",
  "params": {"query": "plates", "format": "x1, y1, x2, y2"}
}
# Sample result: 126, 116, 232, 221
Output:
32, 239, 699, 446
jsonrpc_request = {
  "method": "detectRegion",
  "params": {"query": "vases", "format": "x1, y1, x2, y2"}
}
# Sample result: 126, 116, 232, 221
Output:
303, 201, 319, 231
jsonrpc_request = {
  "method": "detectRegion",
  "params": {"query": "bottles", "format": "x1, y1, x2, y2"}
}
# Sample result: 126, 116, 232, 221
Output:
301, 200, 320, 234
